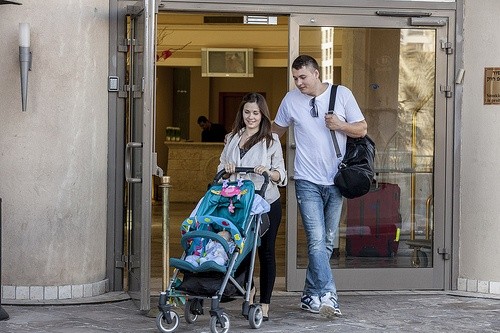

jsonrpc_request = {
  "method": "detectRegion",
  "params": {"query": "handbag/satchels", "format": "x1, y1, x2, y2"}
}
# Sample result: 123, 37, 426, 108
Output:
334, 134, 376, 200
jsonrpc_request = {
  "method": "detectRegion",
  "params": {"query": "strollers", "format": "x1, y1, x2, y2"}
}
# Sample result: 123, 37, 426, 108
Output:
156, 167, 270, 333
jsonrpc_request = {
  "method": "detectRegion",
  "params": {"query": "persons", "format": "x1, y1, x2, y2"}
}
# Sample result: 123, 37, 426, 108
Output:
223, 55, 368, 320
185, 230, 234, 266
217, 93, 288, 321
198, 116, 227, 142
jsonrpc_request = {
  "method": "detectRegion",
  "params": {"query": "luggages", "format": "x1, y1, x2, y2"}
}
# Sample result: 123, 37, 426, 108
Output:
345, 171, 402, 257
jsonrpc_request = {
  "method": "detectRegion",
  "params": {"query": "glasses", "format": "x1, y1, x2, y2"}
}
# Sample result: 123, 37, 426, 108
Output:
309, 96, 318, 117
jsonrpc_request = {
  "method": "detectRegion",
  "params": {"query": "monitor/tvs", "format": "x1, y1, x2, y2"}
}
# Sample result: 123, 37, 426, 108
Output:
200, 48, 254, 77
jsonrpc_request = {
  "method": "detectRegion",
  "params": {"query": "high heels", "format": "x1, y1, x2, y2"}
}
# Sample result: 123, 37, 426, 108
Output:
262, 306, 271, 321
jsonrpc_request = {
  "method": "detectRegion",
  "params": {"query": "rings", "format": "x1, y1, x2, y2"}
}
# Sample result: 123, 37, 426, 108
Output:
227, 167, 230, 170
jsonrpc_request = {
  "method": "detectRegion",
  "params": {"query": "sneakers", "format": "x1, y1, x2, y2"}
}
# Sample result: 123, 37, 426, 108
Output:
318, 293, 342, 322
300, 294, 321, 313
